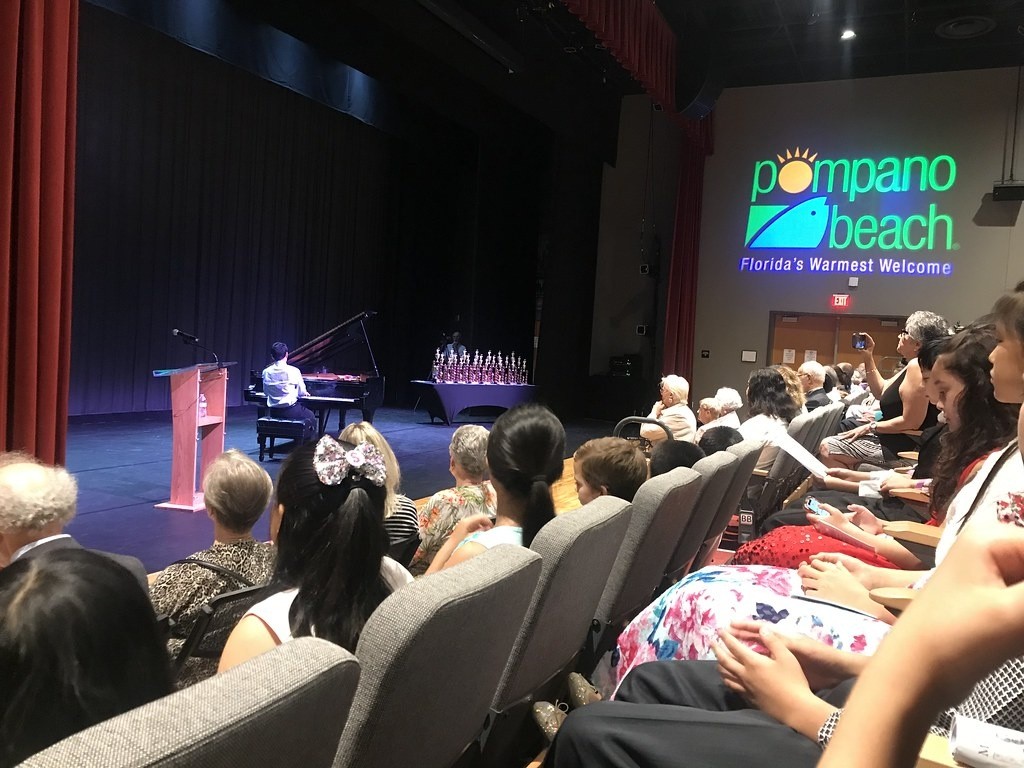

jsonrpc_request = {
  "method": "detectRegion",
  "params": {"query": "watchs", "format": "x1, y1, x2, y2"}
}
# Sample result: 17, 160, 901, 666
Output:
870, 421, 877, 434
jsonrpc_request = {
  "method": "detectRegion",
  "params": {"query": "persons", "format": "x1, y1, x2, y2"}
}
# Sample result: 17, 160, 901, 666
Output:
262, 342, 316, 439
443, 331, 467, 364
420, 401, 566, 577
531, 279, 1024, 768
338, 421, 419, 546
215, 435, 416, 676
407, 424, 497, 577
149, 447, 279, 692
0, 451, 175, 768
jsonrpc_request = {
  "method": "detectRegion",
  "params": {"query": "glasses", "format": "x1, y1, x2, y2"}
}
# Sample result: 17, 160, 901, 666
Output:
901, 327, 908, 337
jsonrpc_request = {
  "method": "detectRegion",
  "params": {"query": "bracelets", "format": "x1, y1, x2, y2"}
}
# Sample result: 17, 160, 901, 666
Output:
866, 365, 877, 373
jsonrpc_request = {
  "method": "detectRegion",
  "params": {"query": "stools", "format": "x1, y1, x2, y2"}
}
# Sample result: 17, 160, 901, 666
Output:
257, 416, 310, 462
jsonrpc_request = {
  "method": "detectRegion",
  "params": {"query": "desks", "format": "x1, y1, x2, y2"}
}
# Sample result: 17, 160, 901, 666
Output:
410, 380, 540, 425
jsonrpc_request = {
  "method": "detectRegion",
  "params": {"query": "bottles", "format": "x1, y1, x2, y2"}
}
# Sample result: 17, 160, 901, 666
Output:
199, 394, 207, 420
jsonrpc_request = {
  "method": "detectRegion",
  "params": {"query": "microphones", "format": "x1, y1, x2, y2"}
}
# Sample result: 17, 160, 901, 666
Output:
172, 329, 199, 341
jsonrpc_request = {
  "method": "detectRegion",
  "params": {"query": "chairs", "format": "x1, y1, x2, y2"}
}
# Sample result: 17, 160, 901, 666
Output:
170, 582, 272, 668
331, 543, 543, 768
655, 451, 739, 598
11, 636, 361, 768
690, 438, 764, 574
587, 467, 703, 657
475, 495, 634, 763
718, 389, 871, 551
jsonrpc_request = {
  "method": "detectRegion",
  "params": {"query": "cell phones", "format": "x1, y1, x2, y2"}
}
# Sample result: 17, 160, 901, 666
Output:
804, 496, 830, 517
852, 333, 867, 350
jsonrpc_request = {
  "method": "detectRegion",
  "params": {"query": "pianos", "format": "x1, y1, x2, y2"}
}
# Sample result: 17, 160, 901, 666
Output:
242, 311, 385, 444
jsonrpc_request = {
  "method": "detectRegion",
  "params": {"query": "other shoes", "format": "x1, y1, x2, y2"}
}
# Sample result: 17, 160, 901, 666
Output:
568, 672, 602, 710
532, 701, 565, 743
